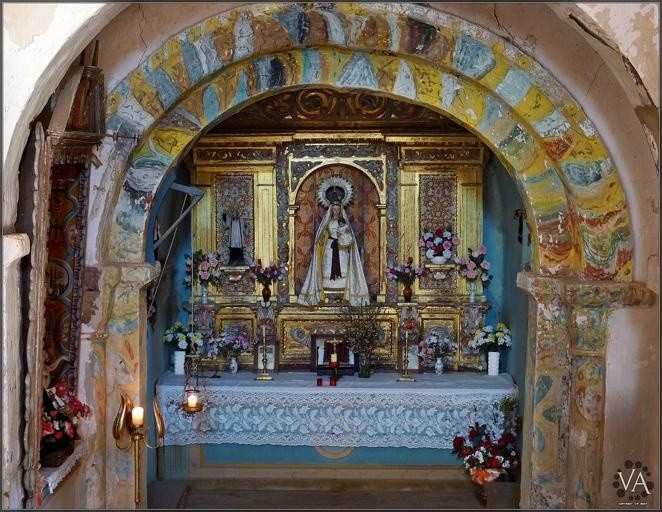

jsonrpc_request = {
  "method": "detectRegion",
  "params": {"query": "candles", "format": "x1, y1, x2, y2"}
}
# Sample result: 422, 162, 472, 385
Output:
330, 376, 336, 386
317, 378, 322, 386
330, 353, 338, 363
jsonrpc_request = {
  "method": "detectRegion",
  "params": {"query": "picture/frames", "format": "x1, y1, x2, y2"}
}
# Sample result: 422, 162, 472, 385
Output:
398, 341, 420, 373
310, 334, 361, 373
254, 340, 279, 373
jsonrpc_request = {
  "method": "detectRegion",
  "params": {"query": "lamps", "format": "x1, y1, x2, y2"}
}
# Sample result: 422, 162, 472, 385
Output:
180, 348, 209, 416
113, 391, 165, 510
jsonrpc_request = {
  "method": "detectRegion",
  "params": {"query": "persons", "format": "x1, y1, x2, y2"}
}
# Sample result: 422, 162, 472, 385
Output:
334, 217, 354, 247
222, 209, 250, 264
303, 192, 370, 306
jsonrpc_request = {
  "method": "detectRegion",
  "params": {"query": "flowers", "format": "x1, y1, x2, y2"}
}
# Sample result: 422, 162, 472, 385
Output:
386, 226, 494, 285
185, 250, 279, 286
41, 382, 95, 439
163, 322, 251, 357
453, 424, 518, 485
341, 301, 512, 357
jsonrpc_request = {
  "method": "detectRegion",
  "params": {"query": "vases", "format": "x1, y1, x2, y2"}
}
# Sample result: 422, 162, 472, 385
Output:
229, 357, 238, 374
40, 432, 75, 468
435, 357, 444, 375
174, 351, 185, 375
358, 352, 371, 378
469, 474, 504, 508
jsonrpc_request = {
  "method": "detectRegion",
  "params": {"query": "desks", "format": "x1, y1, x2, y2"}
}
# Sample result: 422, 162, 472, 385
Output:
155, 367, 516, 484
